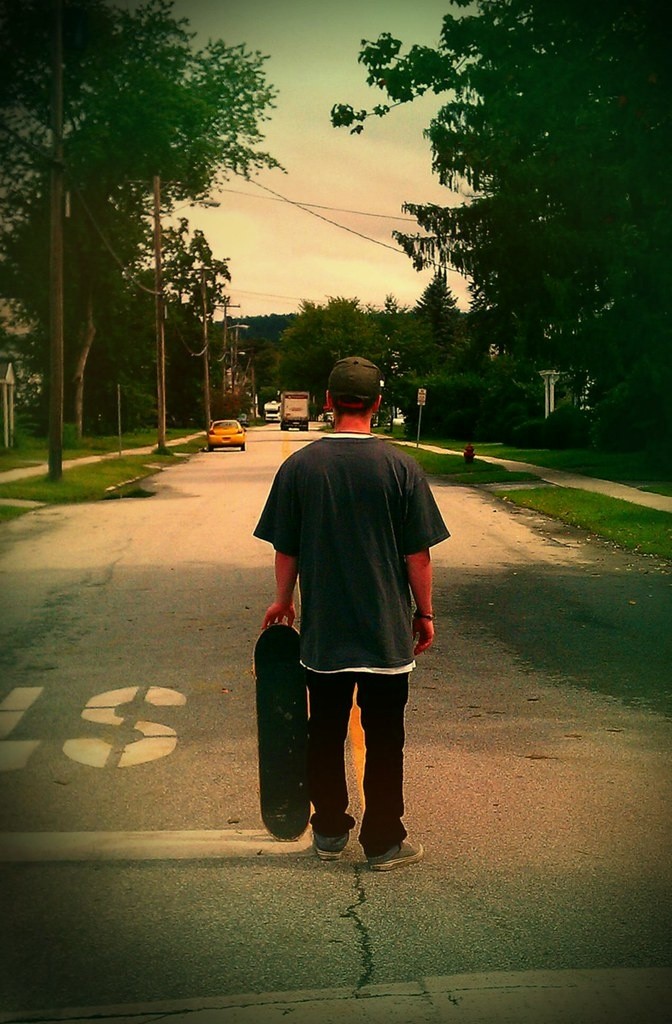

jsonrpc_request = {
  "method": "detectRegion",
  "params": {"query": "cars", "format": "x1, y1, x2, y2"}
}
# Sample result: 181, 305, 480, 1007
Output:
208, 420, 246, 451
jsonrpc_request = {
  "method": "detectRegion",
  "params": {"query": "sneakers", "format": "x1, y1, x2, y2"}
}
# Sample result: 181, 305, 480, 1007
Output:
313, 832, 350, 860
368, 841, 424, 871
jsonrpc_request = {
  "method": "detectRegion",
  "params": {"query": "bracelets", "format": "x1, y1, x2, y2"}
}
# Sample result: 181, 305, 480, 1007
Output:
413, 608, 433, 620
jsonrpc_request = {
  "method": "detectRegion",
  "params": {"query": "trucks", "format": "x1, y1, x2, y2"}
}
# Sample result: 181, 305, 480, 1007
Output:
264, 401, 281, 423
281, 391, 308, 431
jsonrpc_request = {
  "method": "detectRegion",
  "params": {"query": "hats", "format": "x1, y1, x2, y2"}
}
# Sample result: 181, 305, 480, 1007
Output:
328, 356, 385, 408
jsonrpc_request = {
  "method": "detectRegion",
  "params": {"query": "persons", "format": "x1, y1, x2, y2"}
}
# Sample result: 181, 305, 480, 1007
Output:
253, 356, 451, 872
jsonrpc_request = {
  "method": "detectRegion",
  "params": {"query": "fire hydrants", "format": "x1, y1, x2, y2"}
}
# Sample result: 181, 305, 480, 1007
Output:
464, 444, 475, 462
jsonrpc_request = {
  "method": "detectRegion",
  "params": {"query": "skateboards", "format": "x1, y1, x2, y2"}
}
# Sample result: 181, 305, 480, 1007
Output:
254, 624, 312, 843
